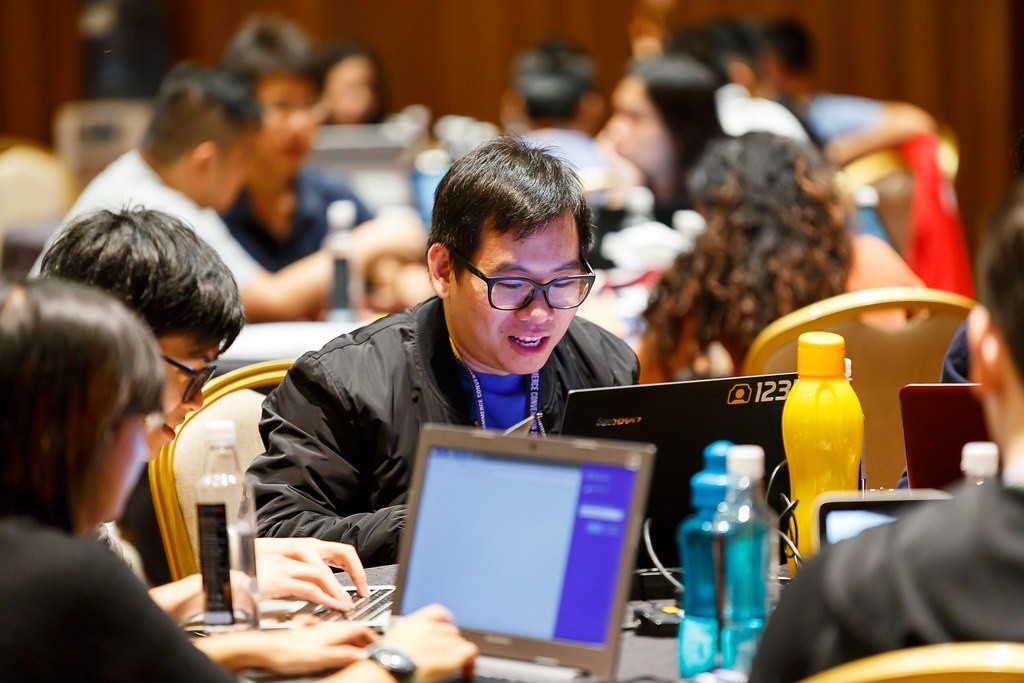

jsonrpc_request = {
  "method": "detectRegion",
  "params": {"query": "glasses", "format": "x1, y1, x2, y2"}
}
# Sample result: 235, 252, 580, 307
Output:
141, 413, 178, 455
453, 248, 597, 311
159, 352, 218, 406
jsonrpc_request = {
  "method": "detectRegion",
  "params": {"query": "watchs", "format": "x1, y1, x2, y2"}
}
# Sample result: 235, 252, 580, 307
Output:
369, 649, 416, 683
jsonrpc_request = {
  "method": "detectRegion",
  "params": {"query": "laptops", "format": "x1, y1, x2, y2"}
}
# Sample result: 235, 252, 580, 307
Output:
198, 371, 995, 682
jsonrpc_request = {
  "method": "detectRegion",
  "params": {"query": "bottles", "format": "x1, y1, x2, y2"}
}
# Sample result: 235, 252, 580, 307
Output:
679, 443, 774, 682
316, 200, 367, 335
617, 189, 654, 315
193, 419, 262, 635
959, 440, 1000, 489
781, 331, 865, 581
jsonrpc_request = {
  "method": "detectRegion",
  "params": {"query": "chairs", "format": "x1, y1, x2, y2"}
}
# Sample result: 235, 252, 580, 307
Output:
148, 358, 297, 582
737, 286, 978, 490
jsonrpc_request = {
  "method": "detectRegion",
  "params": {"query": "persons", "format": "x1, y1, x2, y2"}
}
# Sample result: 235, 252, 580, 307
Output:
0, 2, 1024, 683
